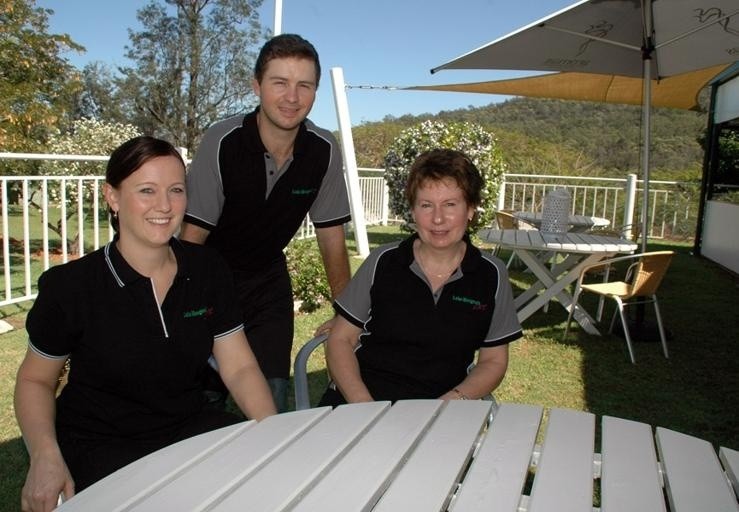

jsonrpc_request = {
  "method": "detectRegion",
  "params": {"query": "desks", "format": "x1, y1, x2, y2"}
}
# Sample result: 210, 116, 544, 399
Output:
57, 394, 739, 512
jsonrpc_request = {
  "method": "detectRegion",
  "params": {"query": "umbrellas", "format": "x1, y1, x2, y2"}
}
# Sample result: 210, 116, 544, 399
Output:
430, 1, 739, 321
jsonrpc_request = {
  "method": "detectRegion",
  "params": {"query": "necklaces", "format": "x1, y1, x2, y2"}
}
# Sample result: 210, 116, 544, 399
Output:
417, 244, 465, 278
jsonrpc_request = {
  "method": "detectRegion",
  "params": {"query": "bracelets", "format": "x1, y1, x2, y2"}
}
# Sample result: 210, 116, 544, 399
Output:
451, 388, 469, 401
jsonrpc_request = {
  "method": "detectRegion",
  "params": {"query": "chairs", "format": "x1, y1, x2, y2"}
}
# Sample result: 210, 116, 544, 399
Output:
292, 331, 499, 428
478, 209, 639, 339
52, 355, 221, 508
562, 249, 676, 364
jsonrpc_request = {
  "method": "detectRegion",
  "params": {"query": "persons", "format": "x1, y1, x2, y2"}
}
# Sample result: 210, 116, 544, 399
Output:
314, 147, 524, 408
13, 135, 280, 512
179, 34, 352, 421
